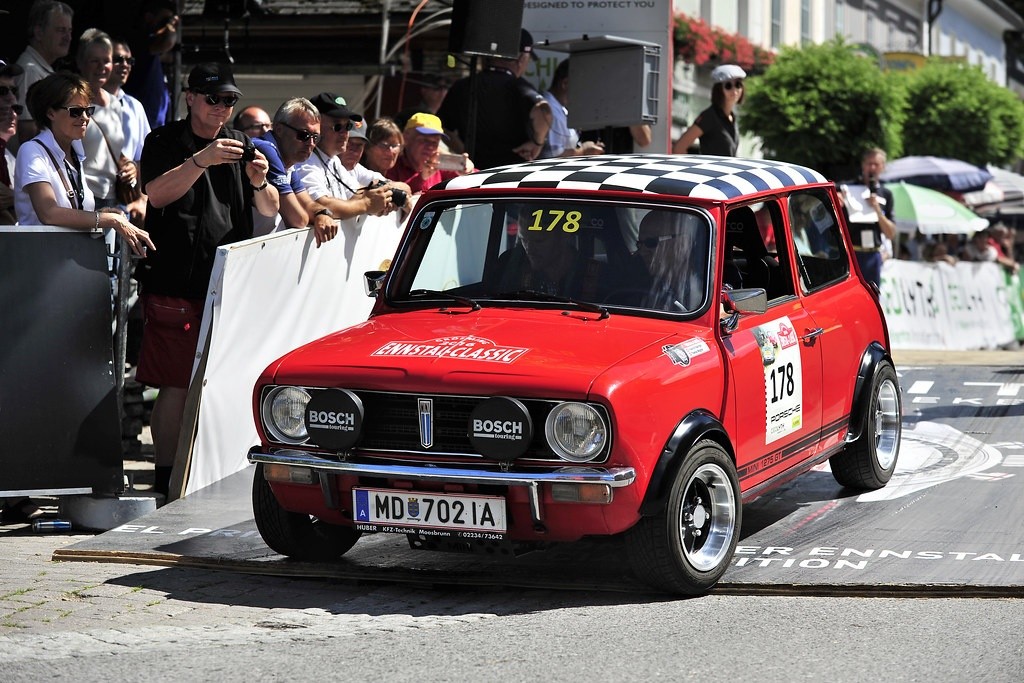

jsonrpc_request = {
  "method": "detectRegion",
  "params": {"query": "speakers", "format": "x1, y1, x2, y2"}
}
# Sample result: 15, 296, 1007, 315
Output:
447, 0, 525, 60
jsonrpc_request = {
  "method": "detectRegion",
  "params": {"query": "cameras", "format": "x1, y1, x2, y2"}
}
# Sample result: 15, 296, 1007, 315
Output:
233, 144, 256, 161
369, 180, 408, 208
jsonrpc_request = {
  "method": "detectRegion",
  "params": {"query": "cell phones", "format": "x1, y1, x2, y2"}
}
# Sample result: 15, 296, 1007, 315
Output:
436, 153, 468, 172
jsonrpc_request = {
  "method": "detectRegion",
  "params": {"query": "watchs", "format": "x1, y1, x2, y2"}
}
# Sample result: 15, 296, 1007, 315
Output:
315, 209, 333, 218
250, 179, 268, 191
532, 136, 547, 146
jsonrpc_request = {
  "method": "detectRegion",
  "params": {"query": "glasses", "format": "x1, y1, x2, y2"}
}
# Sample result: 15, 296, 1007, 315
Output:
0, 103, 23, 115
720, 81, 742, 90
279, 122, 321, 143
197, 92, 239, 108
58, 105, 96, 118
370, 140, 402, 150
0, 85, 19, 97
241, 122, 274, 132
321, 120, 353, 132
112, 55, 135, 65
636, 232, 681, 251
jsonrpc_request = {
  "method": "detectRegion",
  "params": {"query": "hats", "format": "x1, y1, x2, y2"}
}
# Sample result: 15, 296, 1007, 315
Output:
710, 64, 747, 84
0, 60, 24, 78
309, 92, 362, 122
187, 64, 244, 96
348, 117, 370, 143
404, 112, 448, 140
518, 27, 539, 62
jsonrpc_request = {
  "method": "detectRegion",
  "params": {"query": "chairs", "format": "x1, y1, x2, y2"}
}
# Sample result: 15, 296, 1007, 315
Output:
723, 206, 786, 298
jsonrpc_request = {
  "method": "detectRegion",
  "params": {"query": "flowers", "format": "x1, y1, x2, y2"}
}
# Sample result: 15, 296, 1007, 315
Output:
673, 10, 770, 73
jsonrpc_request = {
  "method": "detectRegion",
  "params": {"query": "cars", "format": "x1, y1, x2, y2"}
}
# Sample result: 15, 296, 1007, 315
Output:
246, 153, 902, 596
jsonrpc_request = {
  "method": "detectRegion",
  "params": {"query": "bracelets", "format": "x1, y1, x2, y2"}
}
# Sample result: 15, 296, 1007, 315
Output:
124, 160, 137, 169
94, 211, 99, 232
108, 207, 112, 213
192, 153, 208, 168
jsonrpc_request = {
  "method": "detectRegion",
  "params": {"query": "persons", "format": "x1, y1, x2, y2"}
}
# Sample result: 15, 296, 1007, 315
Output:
436, 28, 651, 253
672, 64, 746, 157
233, 105, 273, 138
294, 93, 480, 219
489, 206, 608, 303
121, 0, 177, 130
139, 62, 281, 507
0, 0, 152, 370
606, 210, 702, 312
252, 97, 338, 248
395, 71, 453, 132
899, 224, 1024, 351
756, 143, 896, 300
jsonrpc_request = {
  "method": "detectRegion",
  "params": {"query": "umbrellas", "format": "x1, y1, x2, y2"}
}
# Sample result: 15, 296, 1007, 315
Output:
877, 154, 1024, 214
